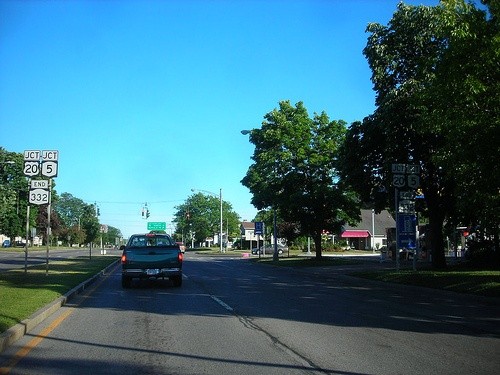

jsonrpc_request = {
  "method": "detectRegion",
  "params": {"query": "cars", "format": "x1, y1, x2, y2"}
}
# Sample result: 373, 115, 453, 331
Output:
252, 243, 289, 255
379, 244, 427, 261
2, 239, 26, 248
119, 232, 185, 290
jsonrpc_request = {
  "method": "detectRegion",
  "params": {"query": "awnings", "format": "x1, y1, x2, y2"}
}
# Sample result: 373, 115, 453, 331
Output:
340, 230, 372, 237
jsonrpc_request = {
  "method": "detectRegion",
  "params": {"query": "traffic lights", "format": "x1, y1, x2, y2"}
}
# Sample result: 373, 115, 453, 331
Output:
96, 207, 100, 215
146, 209, 150, 218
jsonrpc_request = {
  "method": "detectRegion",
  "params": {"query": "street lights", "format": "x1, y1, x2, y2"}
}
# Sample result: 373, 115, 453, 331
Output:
191, 188, 223, 251
